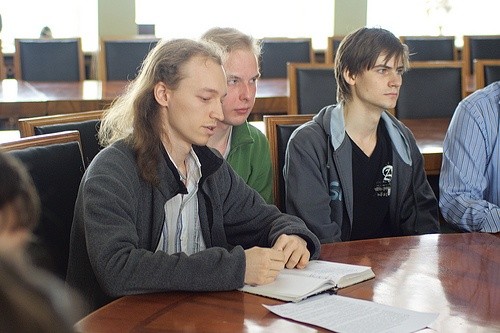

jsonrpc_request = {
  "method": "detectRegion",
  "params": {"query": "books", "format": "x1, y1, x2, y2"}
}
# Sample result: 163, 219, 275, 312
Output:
237, 259, 376, 303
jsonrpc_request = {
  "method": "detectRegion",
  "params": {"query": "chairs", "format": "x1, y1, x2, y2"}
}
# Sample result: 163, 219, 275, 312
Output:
0, 35, 500, 279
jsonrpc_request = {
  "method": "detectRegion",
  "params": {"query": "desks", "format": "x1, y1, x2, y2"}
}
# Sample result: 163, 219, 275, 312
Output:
0, 78, 290, 130
71, 233, 500, 333
397, 114, 452, 176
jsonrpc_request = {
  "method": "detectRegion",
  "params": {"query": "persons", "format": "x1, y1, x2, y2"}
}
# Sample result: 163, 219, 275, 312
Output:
202, 28, 275, 203
285, 28, 439, 243
1, 153, 78, 332
440, 81, 498, 233
67, 39, 322, 308
